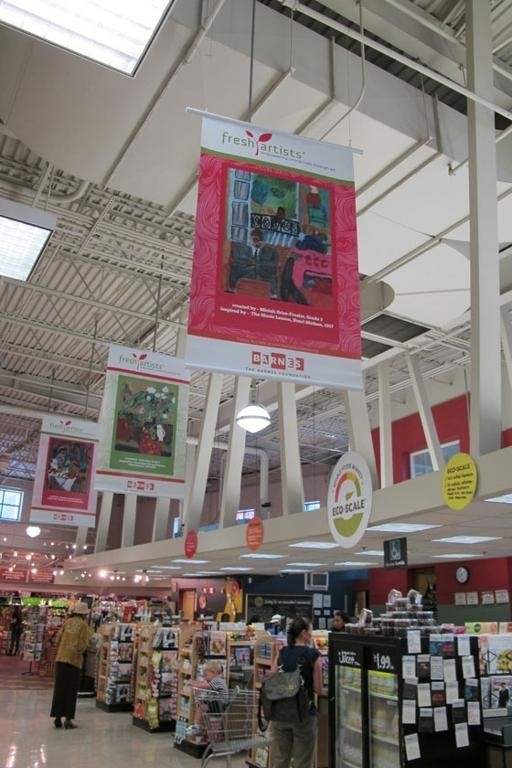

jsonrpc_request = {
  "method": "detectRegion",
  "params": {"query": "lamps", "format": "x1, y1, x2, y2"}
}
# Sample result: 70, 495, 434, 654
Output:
26, 525, 41, 538
236, 379, 272, 434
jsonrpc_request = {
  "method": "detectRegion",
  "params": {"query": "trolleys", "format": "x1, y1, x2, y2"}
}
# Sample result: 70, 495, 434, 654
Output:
189, 685, 287, 768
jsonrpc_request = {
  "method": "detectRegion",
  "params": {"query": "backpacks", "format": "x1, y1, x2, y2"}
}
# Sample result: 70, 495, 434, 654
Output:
258, 646, 320, 732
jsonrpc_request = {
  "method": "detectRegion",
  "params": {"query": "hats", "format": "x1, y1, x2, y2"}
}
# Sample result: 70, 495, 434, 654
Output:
73, 602, 91, 615
270, 614, 282, 623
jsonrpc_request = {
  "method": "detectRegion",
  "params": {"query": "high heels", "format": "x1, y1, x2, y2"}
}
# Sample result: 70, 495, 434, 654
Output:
64, 720, 78, 729
55, 719, 61, 728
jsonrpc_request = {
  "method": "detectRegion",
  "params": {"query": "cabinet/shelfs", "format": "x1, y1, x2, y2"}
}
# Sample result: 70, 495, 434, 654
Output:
246, 638, 329, 768
130, 623, 180, 734
17, 605, 68, 675
96, 623, 135, 713
172, 627, 255, 759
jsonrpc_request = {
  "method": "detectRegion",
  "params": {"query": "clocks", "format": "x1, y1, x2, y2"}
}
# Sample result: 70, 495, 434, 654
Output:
455, 566, 469, 584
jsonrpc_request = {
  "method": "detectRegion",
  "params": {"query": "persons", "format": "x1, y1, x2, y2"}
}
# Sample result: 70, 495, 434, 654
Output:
7, 604, 22, 657
330, 610, 350, 634
498, 682, 510, 709
267, 614, 284, 638
49, 603, 91, 729
240, 228, 278, 292
185, 661, 230, 736
271, 619, 323, 768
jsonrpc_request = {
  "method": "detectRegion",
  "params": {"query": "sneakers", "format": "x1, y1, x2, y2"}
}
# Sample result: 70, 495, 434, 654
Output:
186, 725, 201, 735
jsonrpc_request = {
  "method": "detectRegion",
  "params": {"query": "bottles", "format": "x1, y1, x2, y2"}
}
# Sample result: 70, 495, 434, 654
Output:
339, 665, 400, 768
